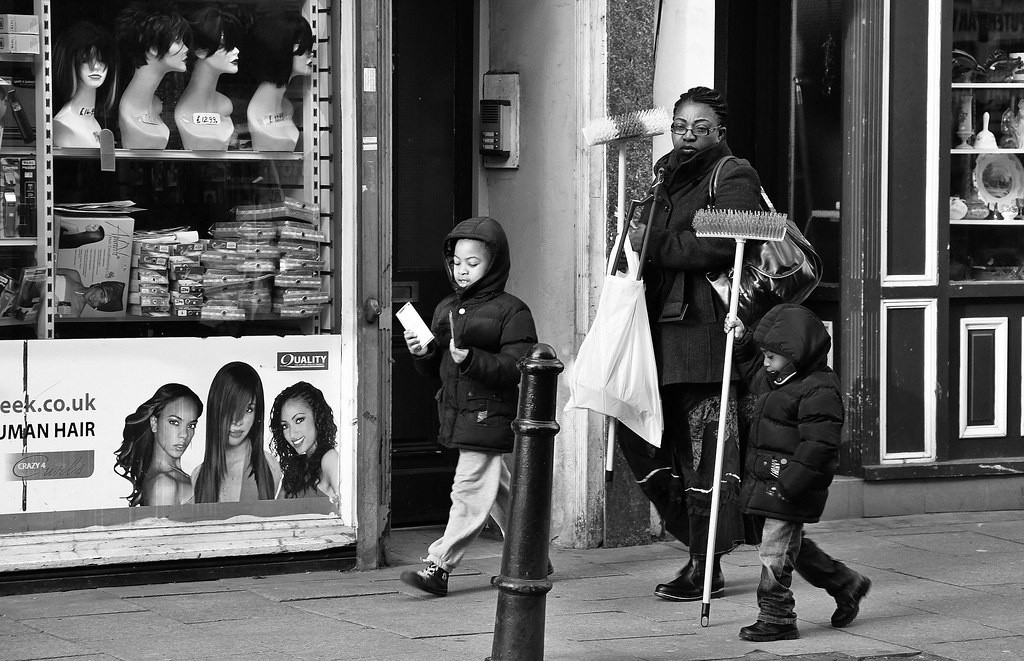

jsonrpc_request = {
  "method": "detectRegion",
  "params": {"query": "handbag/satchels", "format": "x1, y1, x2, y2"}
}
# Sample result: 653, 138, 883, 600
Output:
563, 233, 664, 447
705, 156, 823, 327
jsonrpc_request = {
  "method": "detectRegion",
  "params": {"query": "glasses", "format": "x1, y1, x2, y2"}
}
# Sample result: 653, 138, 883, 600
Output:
671, 121, 722, 136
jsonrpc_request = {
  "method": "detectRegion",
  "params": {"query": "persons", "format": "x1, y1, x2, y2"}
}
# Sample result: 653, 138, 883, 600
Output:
269, 381, 340, 498
116, 4, 314, 152
401, 217, 554, 596
52, 19, 120, 149
191, 362, 283, 503
723, 305, 871, 642
55, 268, 125, 319
113, 382, 204, 506
59, 220, 104, 249
616, 86, 766, 600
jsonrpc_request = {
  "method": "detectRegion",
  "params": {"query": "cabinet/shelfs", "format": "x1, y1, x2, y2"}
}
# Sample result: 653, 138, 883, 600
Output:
0, 0, 335, 340
949, 83, 1024, 297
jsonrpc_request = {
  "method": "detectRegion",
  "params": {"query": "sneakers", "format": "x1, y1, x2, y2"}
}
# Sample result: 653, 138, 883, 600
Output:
489, 556, 554, 590
400, 563, 448, 596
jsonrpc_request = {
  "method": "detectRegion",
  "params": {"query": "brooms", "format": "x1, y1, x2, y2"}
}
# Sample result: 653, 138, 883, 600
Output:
690, 208, 788, 629
581, 107, 674, 490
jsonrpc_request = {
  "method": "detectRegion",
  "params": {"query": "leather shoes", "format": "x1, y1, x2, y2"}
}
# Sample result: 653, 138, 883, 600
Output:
740, 618, 801, 642
655, 553, 726, 601
830, 576, 871, 627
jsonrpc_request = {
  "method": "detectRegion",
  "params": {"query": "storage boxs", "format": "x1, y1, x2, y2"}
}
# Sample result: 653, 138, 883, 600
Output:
1, 15, 330, 322
